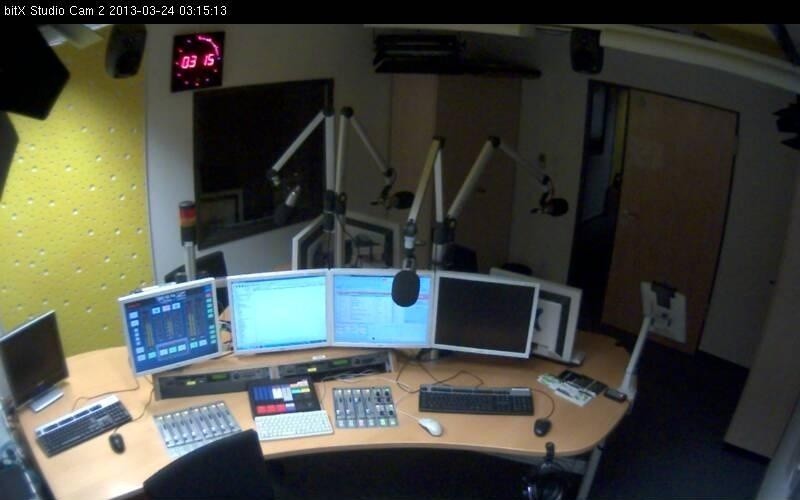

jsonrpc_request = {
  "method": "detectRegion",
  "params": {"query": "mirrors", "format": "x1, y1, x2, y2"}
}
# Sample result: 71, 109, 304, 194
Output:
193, 80, 334, 249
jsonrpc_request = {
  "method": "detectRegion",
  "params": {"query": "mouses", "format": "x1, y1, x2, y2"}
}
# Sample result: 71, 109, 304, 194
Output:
108, 433, 125, 453
534, 418, 551, 435
418, 417, 443, 436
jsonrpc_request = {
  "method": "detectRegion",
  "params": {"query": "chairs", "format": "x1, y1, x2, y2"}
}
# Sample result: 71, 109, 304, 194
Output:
143, 430, 273, 499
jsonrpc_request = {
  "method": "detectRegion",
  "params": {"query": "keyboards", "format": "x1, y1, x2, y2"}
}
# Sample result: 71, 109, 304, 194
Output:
418, 384, 534, 416
571, 350, 587, 365
33, 394, 133, 459
254, 409, 334, 441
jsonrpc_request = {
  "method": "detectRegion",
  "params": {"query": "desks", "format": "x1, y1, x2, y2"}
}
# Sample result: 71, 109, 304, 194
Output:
12, 330, 640, 499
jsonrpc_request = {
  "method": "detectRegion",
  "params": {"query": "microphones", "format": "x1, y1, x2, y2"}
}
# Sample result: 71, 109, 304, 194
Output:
546, 198, 570, 216
391, 255, 420, 308
391, 191, 415, 210
273, 188, 301, 227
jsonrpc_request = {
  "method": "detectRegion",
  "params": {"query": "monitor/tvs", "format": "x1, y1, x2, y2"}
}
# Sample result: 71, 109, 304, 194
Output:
346, 211, 401, 268
329, 267, 435, 349
289, 214, 329, 271
226, 268, 329, 356
489, 268, 582, 365
0, 309, 69, 411
431, 269, 541, 361
117, 277, 223, 378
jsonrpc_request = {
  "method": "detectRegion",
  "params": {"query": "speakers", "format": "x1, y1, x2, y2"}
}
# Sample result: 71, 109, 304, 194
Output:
569, 27, 604, 76
104, 21, 147, 79
